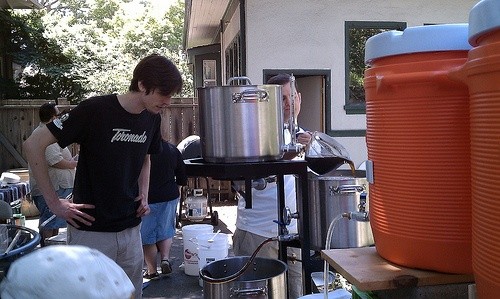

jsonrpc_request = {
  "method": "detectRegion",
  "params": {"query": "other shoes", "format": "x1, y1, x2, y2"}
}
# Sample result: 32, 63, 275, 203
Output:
160, 259, 172, 274
142, 269, 160, 279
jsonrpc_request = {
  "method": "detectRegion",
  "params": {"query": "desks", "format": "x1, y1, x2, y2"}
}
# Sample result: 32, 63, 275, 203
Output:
321, 247, 476, 299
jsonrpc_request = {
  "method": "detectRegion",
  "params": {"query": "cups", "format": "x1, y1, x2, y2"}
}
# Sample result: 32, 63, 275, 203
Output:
0, 225, 9, 254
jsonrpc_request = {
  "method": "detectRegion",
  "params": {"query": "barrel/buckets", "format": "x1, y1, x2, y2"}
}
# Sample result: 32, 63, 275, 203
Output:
465, 1, 500, 299
196, 232, 230, 287
297, 169, 374, 249
195, 76, 285, 164
182, 224, 214, 276
365, 22, 470, 273
199, 256, 288, 299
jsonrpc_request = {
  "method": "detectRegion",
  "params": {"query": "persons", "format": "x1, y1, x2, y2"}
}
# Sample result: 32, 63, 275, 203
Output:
230, 74, 317, 299
24, 53, 189, 299
